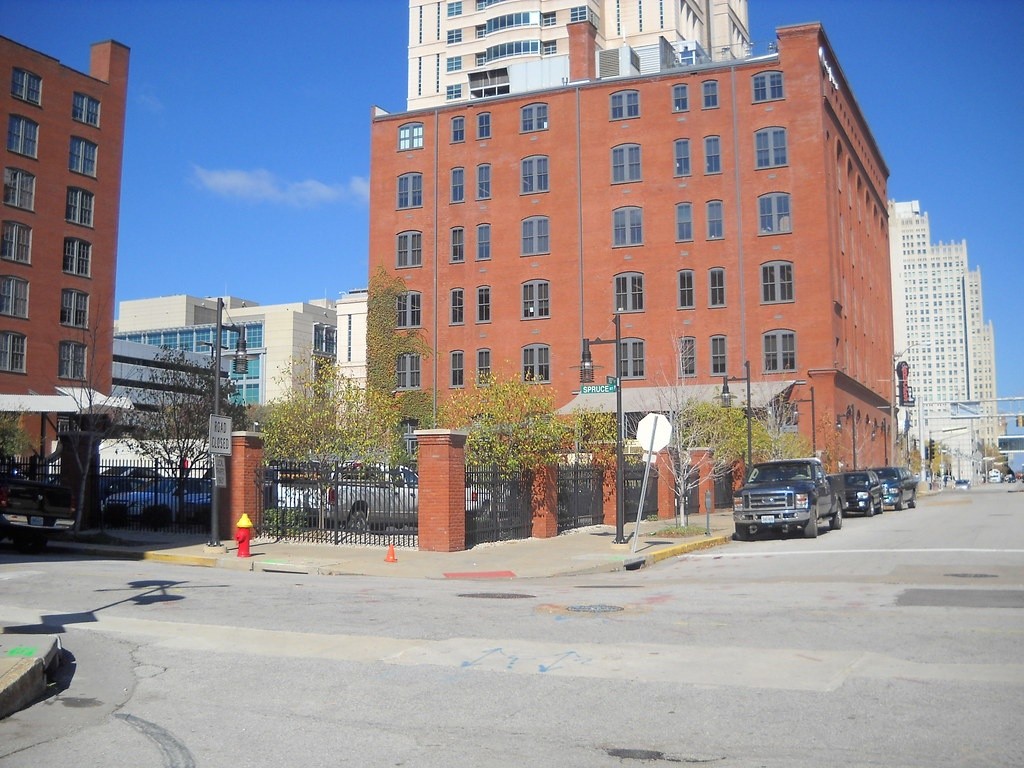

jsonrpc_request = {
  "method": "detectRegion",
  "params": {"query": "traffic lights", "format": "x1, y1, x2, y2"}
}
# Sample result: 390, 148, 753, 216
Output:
925, 447, 929, 460
930, 439, 935, 460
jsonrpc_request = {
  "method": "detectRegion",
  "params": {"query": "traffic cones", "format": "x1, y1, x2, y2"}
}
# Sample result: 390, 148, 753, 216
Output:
384, 543, 398, 563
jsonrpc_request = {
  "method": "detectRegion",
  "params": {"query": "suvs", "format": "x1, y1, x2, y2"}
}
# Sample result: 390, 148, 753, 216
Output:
869, 466, 919, 510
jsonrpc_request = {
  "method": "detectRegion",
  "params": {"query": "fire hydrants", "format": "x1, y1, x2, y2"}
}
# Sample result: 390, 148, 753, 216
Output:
235, 513, 254, 558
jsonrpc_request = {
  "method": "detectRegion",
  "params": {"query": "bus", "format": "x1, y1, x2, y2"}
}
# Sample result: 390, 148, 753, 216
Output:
989, 469, 1002, 483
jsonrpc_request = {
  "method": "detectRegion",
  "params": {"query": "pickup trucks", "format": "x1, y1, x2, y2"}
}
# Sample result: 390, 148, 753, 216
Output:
732, 457, 846, 540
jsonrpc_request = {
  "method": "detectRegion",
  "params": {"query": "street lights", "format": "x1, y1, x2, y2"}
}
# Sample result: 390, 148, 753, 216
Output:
576, 315, 627, 545
790, 387, 817, 458
836, 404, 857, 470
871, 424, 888, 467
719, 360, 752, 468
895, 433, 911, 468
206, 297, 248, 547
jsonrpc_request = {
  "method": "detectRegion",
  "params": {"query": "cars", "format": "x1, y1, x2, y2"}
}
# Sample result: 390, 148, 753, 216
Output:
842, 471, 885, 516
1004, 474, 1016, 483
954, 480, 969, 491
2, 457, 530, 535
